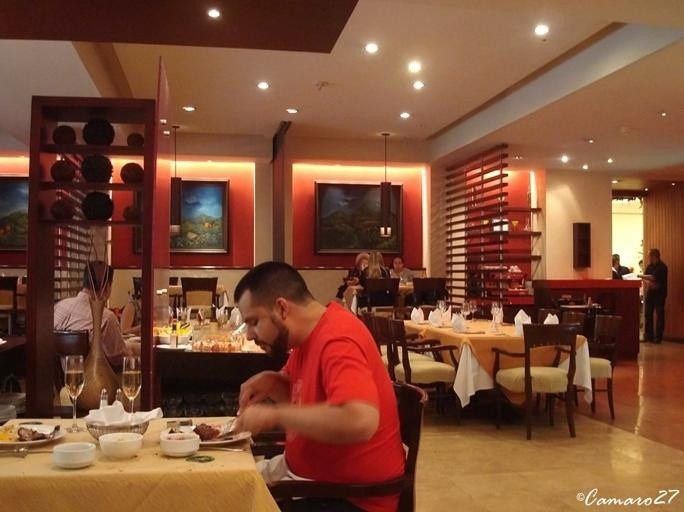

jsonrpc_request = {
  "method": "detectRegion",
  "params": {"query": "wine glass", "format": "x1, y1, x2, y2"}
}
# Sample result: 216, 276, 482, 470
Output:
400, 271, 404, 285
491, 312, 496, 329
436, 300, 447, 317
492, 302, 498, 314
216, 309, 228, 330
460, 303, 470, 320
122, 356, 142, 413
470, 301, 477, 321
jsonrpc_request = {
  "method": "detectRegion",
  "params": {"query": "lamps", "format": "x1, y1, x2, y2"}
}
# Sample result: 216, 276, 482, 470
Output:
169, 126, 185, 226
378, 133, 396, 238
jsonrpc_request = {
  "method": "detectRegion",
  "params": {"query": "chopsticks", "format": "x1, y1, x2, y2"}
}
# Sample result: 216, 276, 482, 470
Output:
86, 421, 150, 441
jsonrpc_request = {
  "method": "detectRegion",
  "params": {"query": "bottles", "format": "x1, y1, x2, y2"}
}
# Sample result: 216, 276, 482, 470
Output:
170, 319, 178, 349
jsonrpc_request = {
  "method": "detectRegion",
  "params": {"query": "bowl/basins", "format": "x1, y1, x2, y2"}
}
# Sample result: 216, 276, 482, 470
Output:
159, 432, 200, 457
159, 335, 189, 344
98, 433, 143, 459
53, 443, 96, 469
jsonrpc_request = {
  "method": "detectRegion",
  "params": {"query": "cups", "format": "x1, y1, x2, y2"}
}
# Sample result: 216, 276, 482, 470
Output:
176, 307, 191, 335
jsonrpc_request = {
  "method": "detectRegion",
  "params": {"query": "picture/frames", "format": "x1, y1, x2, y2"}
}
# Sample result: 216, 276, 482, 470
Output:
313, 180, 405, 256
133, 178, 230, 255
1, 173, 30, 249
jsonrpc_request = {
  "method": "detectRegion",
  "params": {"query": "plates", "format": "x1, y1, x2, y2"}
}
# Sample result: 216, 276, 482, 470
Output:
0, 392, 26, 415
0, 404, 17, 424
0, 340, 8, 345
462, 328, 485, 334
64, 356, 85, 433
155, 345, 189, 350
161, 426, 253, 446
0, 424, 67, 447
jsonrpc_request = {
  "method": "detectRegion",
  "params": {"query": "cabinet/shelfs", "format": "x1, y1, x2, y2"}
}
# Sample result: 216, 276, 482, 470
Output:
470, 207, 543, 259
38, 120, 146, 222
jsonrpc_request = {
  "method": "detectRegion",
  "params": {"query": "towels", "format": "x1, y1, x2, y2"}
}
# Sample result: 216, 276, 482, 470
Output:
229, 308, 242, 326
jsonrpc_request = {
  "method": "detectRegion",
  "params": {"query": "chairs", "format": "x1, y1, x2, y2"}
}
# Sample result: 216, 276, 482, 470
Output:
178, 276, 218, 307
132, 276, 177, 300
360, 306, 616, 439
0, 276, 27, 336
413, 277, 448, 304
363, 278, 399, 305
255, 382, 428, 512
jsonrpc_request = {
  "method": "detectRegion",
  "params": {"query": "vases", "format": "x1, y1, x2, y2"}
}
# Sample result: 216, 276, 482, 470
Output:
69, 299, 118, 411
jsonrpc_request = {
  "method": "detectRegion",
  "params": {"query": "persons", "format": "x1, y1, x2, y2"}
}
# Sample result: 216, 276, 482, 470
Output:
612, 253, 634, 280
390, 256, 413, 283
359, 250, 390, 307
636, 248, 668, 344
335, 251, 370, 300
227, 259, 407, 512
119, 271, 142, 335
52, 259, 142, 375
